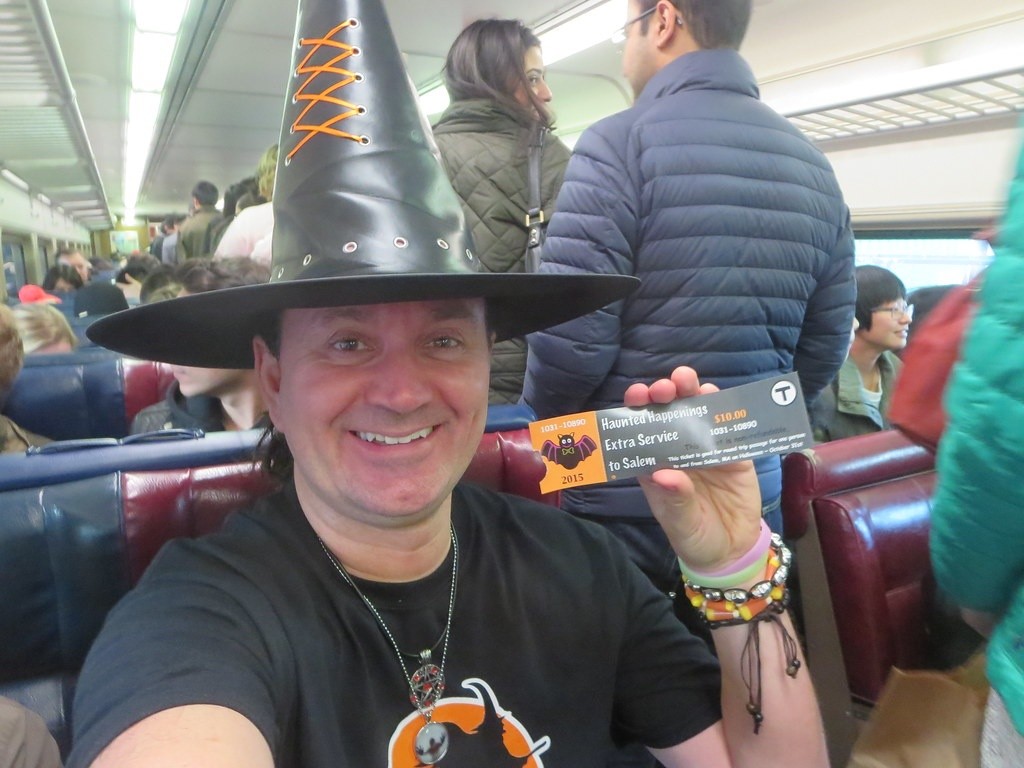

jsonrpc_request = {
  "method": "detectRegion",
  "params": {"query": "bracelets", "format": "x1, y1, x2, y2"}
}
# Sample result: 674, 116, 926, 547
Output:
680, 520, 800, 736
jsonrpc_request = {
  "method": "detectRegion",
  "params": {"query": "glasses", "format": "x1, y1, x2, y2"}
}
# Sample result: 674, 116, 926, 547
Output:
864, 304, 914, 321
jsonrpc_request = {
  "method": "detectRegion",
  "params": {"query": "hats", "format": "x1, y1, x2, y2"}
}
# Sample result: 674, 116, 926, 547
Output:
85, 0, 642, 371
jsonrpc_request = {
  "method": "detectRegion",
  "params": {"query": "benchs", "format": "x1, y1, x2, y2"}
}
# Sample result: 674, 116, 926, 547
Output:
783, 426, 935, 768
0, 265, 565, 766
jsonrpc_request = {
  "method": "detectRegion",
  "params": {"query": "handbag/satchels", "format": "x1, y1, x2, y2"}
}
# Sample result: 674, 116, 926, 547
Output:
847, 647, 987, 768
521, 122, 549, 273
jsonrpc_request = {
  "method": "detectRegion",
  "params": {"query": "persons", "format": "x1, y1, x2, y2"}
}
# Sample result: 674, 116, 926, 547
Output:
431, 0, 856, 652
810, 132, 1024, 768
0, 141, 279, 455
69, 298, 830, 768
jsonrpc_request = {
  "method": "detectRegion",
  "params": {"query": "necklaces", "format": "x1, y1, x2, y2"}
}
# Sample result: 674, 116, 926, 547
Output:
319, 521, 460, 763
